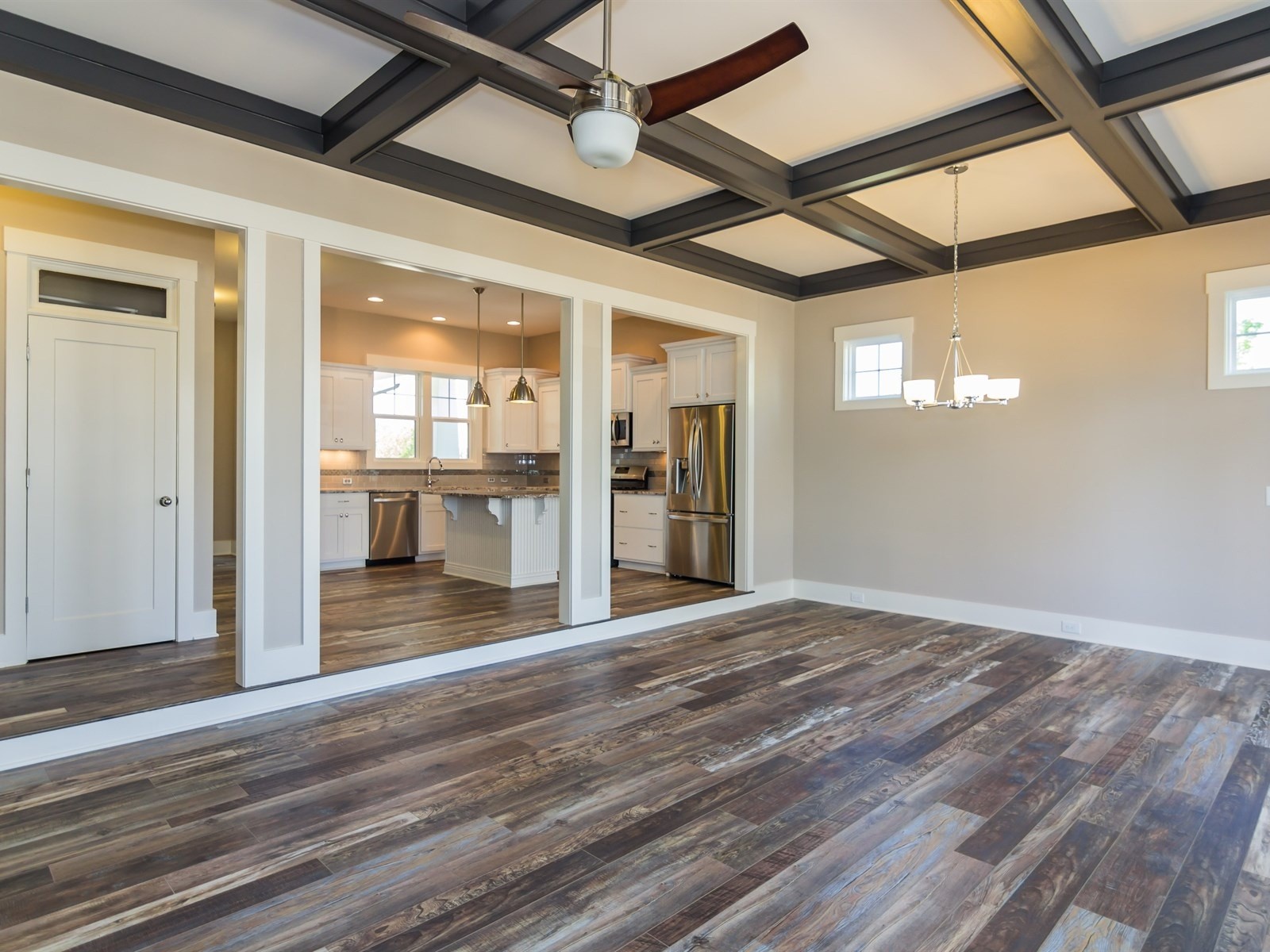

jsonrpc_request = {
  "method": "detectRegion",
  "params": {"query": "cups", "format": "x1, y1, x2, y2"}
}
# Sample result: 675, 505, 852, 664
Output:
514, 454, 540, 476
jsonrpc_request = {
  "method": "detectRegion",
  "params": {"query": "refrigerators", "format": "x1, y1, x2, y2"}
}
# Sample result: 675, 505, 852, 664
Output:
667, 404, 735, 587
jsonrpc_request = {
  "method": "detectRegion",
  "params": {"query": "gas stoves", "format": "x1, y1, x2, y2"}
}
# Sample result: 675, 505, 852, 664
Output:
611, 464, 648, 491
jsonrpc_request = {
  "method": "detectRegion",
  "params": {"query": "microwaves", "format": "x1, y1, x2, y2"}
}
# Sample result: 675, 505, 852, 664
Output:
611, 412, 632, 448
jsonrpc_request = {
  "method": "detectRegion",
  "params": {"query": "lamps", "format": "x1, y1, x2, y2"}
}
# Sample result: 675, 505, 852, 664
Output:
464, 288, 491, 408
902, 165, 1021, 409
572, 111, 640, 167
507, 294, 538, 403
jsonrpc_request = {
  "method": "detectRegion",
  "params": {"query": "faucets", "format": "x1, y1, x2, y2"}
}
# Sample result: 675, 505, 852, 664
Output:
426, 457, 444, 487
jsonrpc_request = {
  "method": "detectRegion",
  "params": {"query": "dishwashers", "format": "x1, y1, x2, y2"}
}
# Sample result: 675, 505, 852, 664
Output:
366, 491, 420, 568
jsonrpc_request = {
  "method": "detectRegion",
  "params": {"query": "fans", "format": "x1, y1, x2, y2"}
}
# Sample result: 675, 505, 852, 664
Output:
402, 0, 808, 144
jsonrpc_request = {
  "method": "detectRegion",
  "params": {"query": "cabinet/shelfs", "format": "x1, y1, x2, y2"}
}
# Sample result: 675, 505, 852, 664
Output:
414, 492, 447, 562
320, 361, 375, 450
320, 491, 369, 571
484, 334, 736, 455
613, 493, 666, 574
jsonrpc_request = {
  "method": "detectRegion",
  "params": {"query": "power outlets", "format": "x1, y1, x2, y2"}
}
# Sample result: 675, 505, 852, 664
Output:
543, 478, 549, 483
488, 477, 495, 482
500, 477, 508, 483
342, 477, 352, 484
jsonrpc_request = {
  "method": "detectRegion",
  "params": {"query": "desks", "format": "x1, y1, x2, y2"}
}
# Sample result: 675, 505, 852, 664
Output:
422, 490, 562, 588
